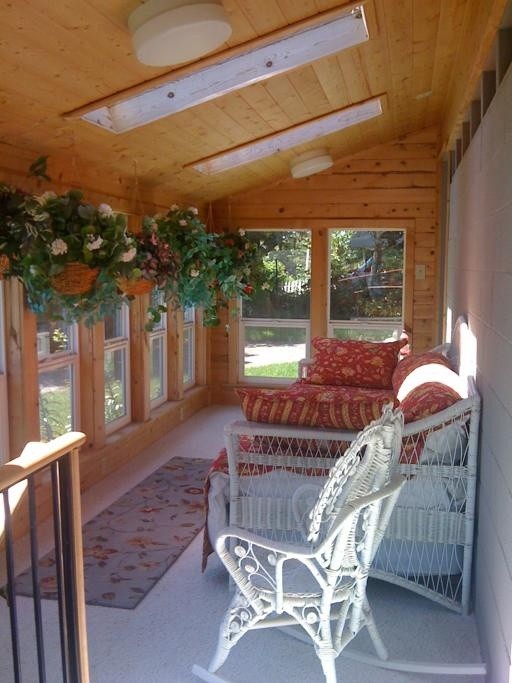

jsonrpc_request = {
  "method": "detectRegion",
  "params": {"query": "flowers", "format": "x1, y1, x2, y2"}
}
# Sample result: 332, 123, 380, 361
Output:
0, 181, 275, 335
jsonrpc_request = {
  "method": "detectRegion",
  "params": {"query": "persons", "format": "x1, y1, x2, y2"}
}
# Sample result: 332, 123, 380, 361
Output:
358, 249, 386, 302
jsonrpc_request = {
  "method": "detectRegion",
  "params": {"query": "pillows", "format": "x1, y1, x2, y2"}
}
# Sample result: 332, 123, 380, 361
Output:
302, 335, 408, 390
233, 383, 395, 452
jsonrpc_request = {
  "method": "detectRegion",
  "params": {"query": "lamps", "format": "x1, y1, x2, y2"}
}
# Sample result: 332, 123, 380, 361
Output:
289, 145, 335, 179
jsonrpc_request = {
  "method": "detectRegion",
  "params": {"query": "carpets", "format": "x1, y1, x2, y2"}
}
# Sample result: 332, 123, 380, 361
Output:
0, 455, 214, 609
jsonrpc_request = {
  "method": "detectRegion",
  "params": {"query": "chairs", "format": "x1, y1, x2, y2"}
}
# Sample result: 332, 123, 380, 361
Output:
191, 403, 489, 683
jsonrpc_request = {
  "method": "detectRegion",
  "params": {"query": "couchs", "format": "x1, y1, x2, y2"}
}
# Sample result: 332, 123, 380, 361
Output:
203, 357, 480, 610
